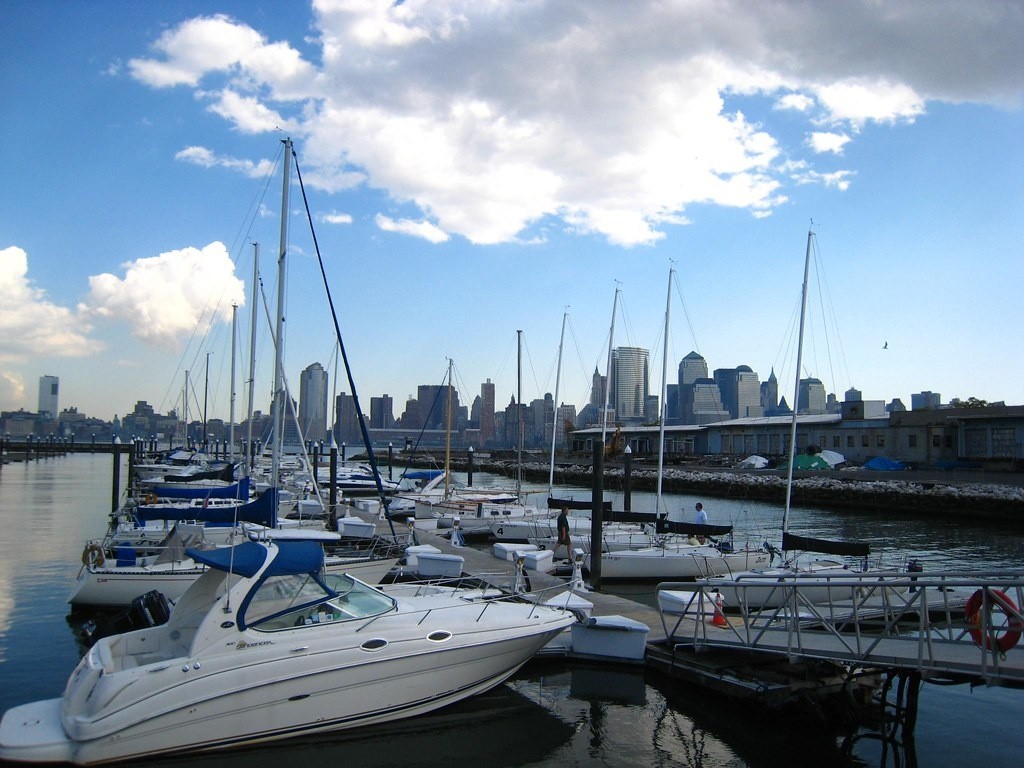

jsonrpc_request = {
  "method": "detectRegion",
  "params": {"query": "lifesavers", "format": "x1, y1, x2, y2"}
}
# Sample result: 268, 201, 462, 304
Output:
966, 590, 1022, 653
145, 493, 159, 505
82, 545, 105, 568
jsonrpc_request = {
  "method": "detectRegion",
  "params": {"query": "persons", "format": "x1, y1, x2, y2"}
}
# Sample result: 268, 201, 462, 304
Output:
550, 507, 574, 564
693, 502, 708, 546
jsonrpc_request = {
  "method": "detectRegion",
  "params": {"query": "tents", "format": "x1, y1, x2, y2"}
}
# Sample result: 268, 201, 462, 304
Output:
732, 448, 985, 471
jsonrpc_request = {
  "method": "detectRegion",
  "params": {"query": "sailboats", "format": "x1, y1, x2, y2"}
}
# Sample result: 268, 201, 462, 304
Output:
1, 104, 1012, 768
